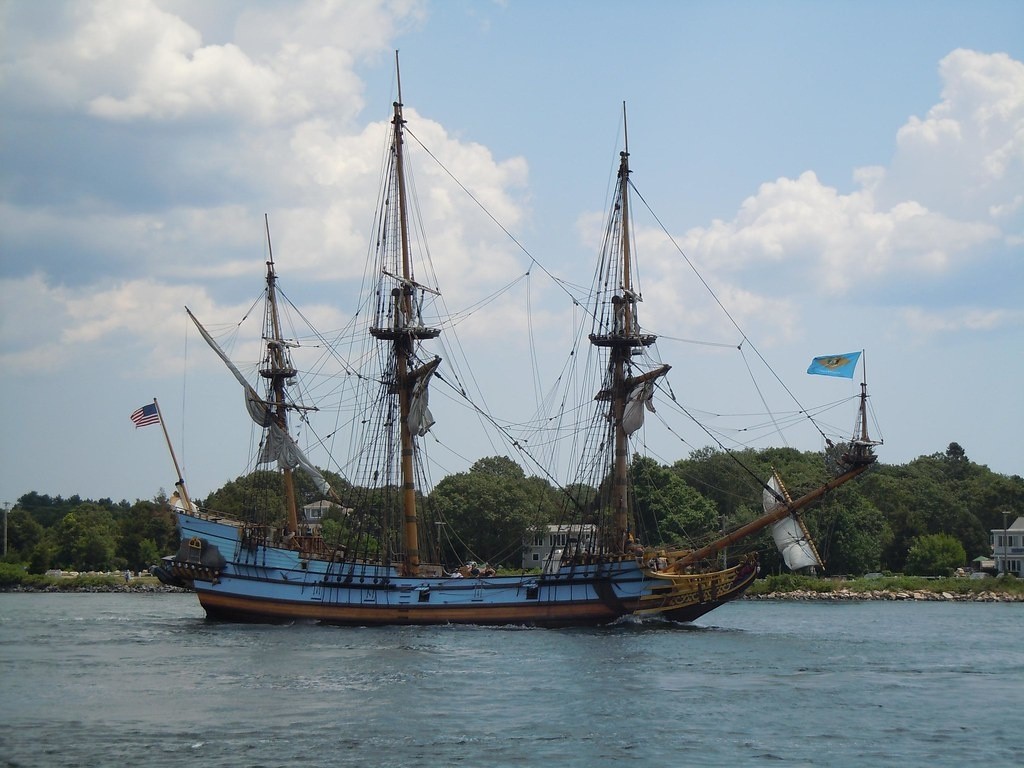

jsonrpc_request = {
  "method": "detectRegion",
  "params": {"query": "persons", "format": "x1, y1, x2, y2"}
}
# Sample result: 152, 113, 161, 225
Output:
451, 567, 463, 577
125, 569, 131, 584
484, 565, 496, 577
584, 533, 591, 552
470, 562, 480, 577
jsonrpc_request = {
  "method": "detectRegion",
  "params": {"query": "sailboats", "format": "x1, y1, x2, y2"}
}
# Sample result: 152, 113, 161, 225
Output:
131, 44, 884, 625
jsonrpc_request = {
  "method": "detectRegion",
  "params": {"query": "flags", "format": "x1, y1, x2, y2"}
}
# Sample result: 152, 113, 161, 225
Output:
807, 351, 862, 378
130, 403, 160, 430
